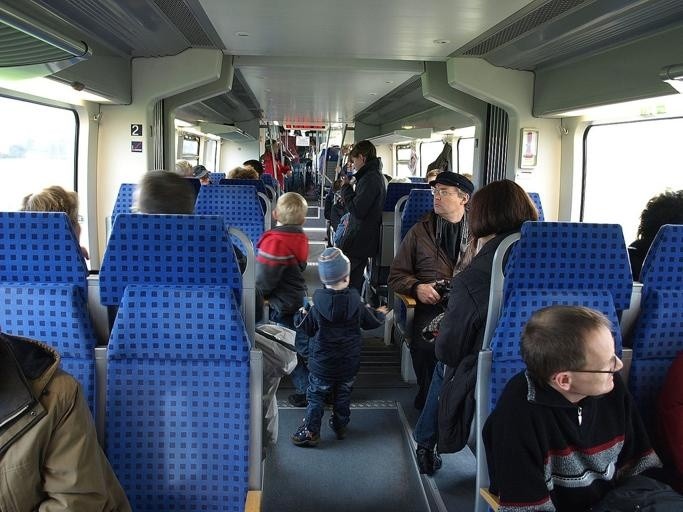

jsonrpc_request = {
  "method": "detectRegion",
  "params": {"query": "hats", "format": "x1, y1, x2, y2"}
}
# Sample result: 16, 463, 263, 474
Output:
319, 248, 351, 284
429, 171, 474, 196
192, 165, 211, 179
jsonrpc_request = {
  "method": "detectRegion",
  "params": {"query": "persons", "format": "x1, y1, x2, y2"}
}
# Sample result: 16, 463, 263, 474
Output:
387, 172, 484, 411
411, 179, 541, 474
291, 248, 386, 446
659, 347, 681, 492
20, 183, 92, 263
637, 189, 681, 282
256, 192, 312, 328
130, 166, 198, 218
174, 138, 445, 293
481, 305, 683, 512
0, 330, 137, 512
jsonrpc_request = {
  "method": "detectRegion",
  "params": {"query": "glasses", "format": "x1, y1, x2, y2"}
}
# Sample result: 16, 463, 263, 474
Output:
554, 355, 617, 383
432, 188, 458, 196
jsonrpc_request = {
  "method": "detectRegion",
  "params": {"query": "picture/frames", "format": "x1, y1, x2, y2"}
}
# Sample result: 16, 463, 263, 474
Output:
518, 126, 540, 170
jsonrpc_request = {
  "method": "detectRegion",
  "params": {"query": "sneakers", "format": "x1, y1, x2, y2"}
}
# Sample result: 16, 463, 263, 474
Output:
328, 417, 347, 439
416, 449, 442, 475
289, 426, 320, 446
289, 394, 307, 408
